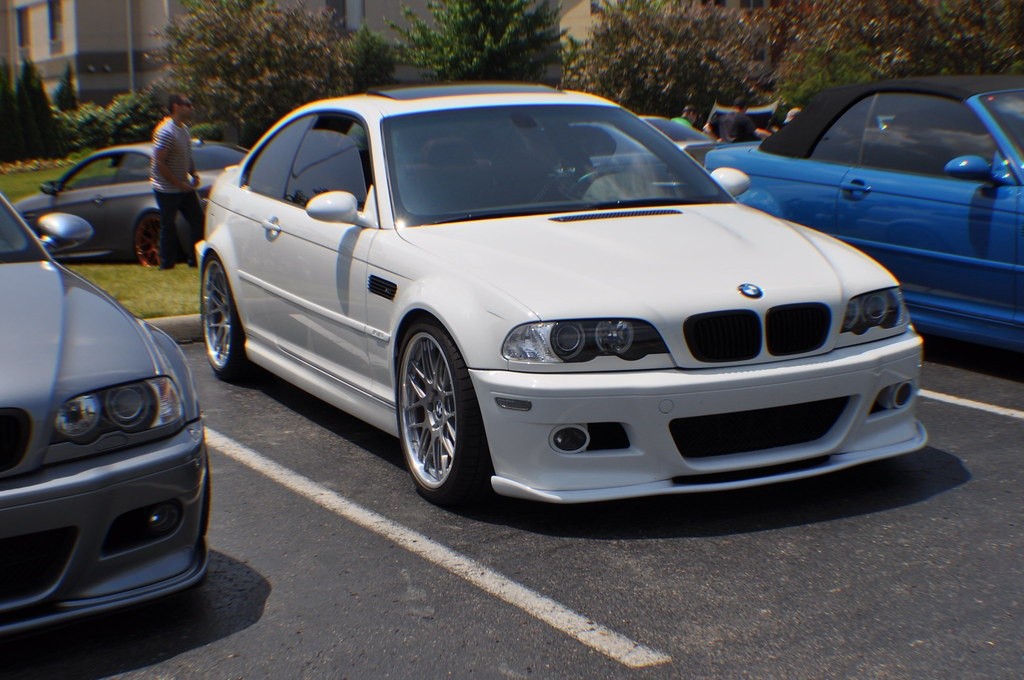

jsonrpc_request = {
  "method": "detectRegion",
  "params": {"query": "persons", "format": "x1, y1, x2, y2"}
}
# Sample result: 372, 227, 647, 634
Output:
149, 90, 205, 270
397, 124, 483, 182
703, 96, 802, 143
670, 104, 698, 127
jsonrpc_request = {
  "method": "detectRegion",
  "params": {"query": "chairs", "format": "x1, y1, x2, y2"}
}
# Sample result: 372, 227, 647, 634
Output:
112, 169, 134, 185
403, 136, 491, 195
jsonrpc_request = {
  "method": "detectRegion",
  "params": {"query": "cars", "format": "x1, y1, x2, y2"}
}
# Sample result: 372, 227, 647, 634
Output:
194, 83, 931, 512
9, 138, 251, 269
701, 73, 1024, 358
562, 116, 724, 203
0, 191, 215, 640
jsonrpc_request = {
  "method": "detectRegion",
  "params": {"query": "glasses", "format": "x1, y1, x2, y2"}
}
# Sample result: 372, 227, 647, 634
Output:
178, 101, 192, 109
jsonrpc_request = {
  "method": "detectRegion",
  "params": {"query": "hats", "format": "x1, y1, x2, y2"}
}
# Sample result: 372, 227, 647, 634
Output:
683, 105, 703, 120
785, 108, 801, 123
